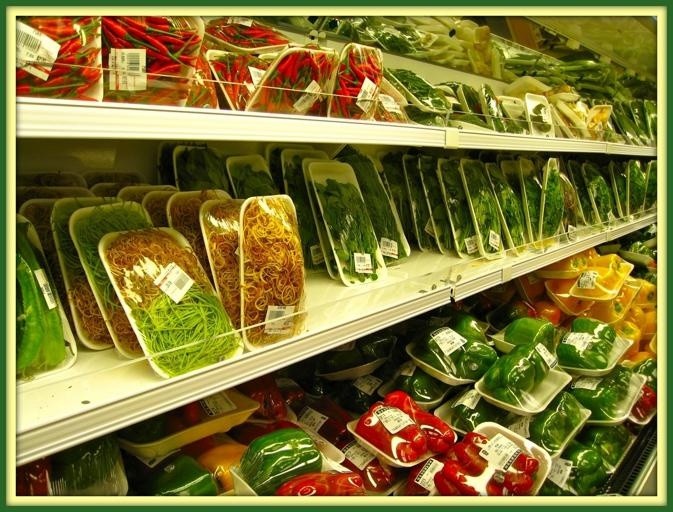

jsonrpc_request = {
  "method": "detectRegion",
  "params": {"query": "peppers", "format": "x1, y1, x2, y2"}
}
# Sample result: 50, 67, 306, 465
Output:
16, 17, 385, 121
16, 223, 657, 496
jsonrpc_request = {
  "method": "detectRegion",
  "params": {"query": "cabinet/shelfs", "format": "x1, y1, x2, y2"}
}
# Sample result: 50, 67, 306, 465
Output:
15, 27, 656, 497
